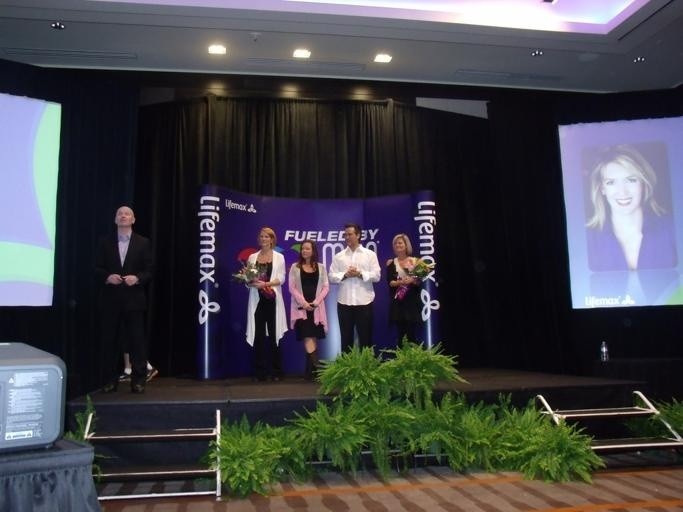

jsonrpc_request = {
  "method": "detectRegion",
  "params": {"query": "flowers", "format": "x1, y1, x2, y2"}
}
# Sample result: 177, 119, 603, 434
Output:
230, 261, 266, 284
404, 256, 437, 279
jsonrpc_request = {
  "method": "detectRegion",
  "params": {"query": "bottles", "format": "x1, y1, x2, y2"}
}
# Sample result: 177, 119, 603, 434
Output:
601, 341, 609, 363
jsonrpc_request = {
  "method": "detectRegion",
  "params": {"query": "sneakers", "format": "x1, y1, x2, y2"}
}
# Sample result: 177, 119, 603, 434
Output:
117, 367, 159, 382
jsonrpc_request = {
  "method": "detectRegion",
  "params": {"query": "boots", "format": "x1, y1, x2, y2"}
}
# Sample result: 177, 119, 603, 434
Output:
252, 374, 281, 384
305, 350, 320, 381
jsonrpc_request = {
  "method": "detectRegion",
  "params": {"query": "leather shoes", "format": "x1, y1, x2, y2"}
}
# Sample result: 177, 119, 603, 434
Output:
130, 381, 146, 393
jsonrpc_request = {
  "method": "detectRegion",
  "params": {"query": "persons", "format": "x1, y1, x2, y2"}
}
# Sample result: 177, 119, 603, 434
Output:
288, 239, 330, 384
386, 233, 421, 348
328, 223, 381, 358
586, 145, 677, 303
245, 228, 288, 383
94, 205, 158, 394
